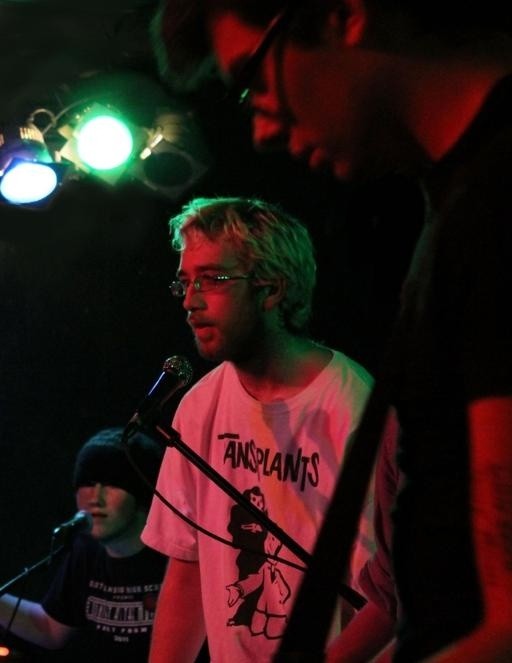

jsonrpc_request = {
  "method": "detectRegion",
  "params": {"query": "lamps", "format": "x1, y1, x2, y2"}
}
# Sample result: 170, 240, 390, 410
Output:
0, 120, 68, 209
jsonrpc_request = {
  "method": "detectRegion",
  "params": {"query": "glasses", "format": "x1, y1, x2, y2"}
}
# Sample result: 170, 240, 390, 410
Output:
220, 11, 290, 116
168, 274, 249, 298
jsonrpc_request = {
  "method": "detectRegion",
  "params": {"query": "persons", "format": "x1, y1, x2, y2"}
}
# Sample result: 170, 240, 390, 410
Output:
1, 426, 169, 655
206, 1, 511, 658
148, 198, 405, 658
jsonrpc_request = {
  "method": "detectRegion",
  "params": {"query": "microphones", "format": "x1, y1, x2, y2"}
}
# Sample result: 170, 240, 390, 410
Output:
51, 509, 94, 539
120, 350, 195, 442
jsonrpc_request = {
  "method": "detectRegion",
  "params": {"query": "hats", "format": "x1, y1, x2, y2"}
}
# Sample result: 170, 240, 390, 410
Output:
71, 425, 159, 494
